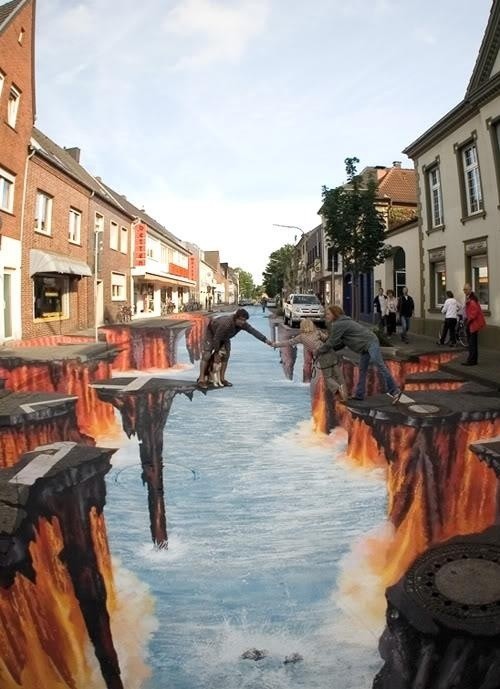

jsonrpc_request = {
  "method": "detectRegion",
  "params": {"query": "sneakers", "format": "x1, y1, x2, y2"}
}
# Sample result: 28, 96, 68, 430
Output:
461, 361, 478, 365
338, 384, 364, 403
391, 388, 403, 406
195, 377, 232, 389
436, 341, 457, 347
387, 332, 409, 344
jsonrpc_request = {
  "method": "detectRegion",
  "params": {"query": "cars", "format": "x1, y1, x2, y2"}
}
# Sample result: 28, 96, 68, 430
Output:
282, 293, 325, 329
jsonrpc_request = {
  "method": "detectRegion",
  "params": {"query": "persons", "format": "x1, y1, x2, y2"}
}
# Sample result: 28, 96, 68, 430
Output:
461, 283, 486, 366
438, 291, 461, 346
276, 296, 279, 303
260, 297, 267, 312
373, 287, 387, 327
385, 290, 399, 336
396, 288, 414, 341
275, 318, 349, 402
196, 308, 272, 388
315, 306, 401, 406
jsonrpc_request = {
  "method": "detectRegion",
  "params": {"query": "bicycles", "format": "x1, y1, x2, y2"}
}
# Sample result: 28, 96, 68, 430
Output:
437, 312, 469, 348
